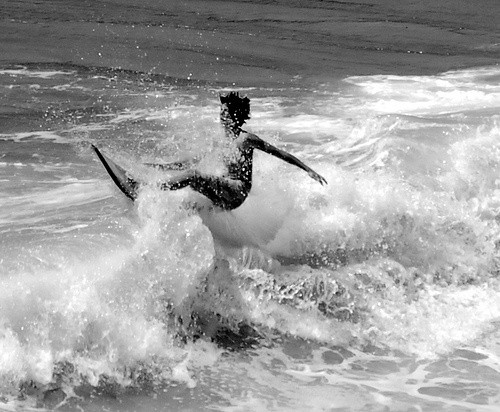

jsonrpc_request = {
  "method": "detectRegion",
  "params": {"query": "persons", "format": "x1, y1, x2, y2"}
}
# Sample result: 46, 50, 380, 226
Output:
142, 90, 327, 210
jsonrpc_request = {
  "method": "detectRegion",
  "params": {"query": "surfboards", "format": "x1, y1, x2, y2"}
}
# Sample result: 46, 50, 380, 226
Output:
90, 144, 137, 201
